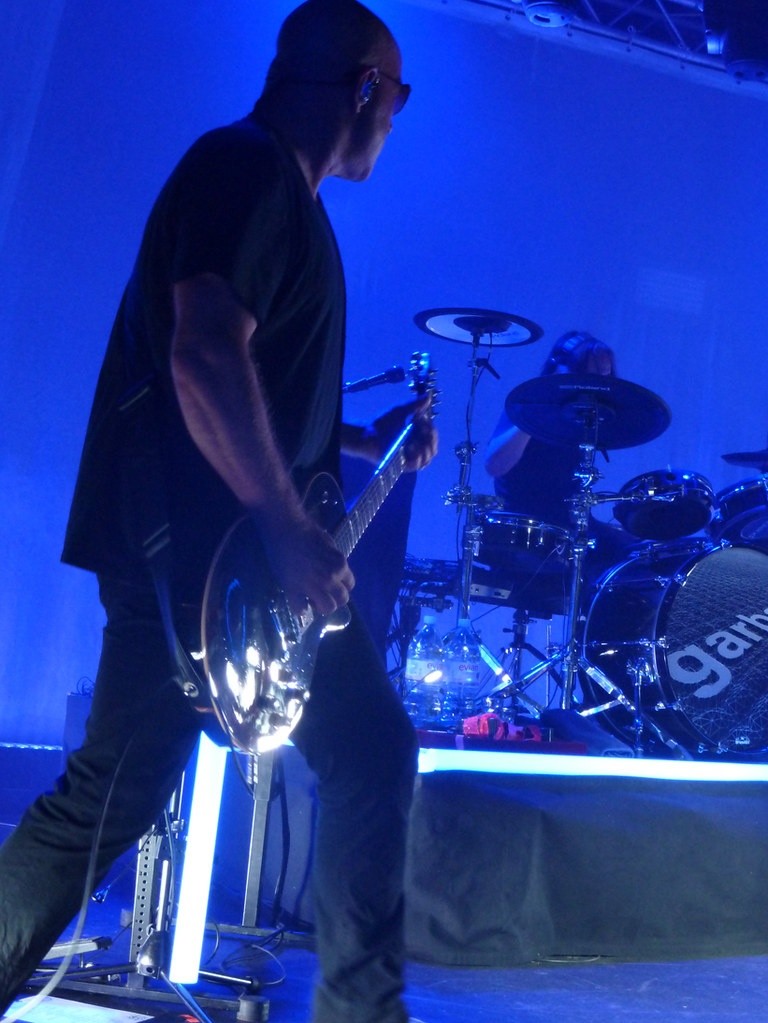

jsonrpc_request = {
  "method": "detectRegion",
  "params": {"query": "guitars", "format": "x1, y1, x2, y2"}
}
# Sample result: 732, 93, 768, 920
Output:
168, 351, 444, 756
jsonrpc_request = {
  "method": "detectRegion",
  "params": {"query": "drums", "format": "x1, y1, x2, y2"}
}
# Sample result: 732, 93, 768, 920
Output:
612, 468, 715, 539
575, 543, 768, 759
705, 473, 768, 542
475, 512, 571, 574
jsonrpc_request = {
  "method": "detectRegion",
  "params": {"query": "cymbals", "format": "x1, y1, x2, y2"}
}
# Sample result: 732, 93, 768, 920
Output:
505, 371, 672, 450
413, 307, 544, 348
721, 449, 768, 471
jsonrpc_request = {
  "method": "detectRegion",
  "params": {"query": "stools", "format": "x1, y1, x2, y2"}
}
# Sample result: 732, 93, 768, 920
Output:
472, 584, 574, 715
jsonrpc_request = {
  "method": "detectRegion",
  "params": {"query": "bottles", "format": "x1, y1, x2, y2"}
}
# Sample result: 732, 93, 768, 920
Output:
403, 616, 443, 729
441, 619, 481, 731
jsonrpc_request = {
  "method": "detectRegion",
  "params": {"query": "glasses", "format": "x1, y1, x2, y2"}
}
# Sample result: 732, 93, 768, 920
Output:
372, 69, 412, 116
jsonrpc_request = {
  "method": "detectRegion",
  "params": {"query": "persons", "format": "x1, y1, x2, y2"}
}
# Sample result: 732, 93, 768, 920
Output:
0, 0, 439, 1023
484, 330, 642, 619
